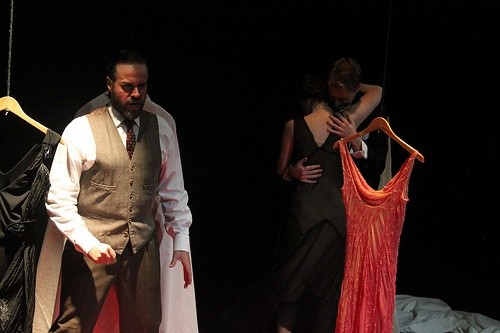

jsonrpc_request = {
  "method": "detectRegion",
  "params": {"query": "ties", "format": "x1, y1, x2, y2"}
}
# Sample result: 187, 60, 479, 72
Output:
123, 118, 136, 161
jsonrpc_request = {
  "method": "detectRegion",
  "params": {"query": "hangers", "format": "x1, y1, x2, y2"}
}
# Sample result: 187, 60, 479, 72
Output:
333, 116, 425, 164
0, 95, 65, 145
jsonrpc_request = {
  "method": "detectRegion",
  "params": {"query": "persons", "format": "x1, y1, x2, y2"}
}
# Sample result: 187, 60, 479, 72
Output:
282, 50, 387, 191
271, 73, 383, 333
44, 48, 192, 333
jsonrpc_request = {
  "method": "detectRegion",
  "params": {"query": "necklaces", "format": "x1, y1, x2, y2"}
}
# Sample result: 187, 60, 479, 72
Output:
311, 106, 334, 116
